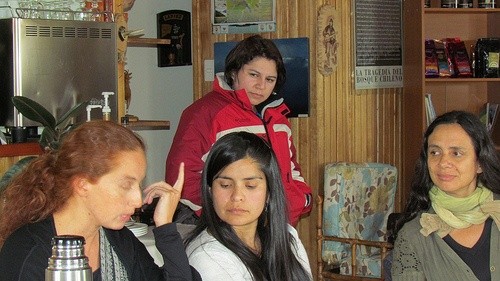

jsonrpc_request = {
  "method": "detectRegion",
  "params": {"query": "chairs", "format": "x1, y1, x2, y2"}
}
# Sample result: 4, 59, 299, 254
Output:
314, 162, 399, 281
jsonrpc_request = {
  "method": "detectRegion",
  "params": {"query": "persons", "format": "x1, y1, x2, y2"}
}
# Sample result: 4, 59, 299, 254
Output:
388, 109, 500, 281
0, 120, 185, 281
164, 35, 316, 281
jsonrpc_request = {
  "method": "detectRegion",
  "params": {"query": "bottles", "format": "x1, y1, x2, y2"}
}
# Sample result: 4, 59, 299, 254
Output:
45, 235, 93, 281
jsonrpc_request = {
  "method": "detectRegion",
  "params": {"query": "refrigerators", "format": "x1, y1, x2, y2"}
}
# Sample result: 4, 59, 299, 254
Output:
1, 18, 119, 127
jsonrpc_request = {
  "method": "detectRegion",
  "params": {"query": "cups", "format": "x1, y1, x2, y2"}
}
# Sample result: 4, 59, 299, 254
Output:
12, 128, 27, 143
26, 127, 38, 137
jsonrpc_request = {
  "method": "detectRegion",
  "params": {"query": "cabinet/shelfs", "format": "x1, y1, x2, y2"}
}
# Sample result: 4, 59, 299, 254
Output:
405, 0, 500, 201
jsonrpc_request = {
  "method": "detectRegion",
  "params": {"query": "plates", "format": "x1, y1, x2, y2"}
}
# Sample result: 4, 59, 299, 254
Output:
124, 223, 148, 237
